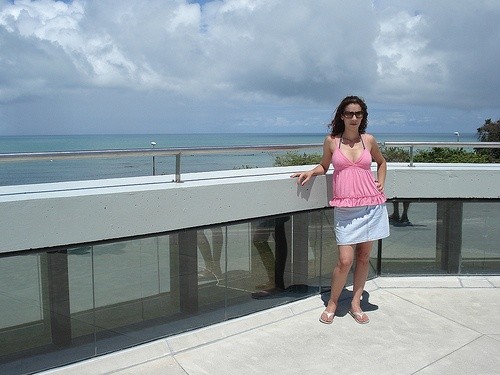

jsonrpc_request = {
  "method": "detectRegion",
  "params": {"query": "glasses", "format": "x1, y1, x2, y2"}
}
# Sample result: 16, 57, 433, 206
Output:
342, 110, 364, 119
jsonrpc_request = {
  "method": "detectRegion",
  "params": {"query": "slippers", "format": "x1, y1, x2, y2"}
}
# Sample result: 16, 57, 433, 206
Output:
319, 309, 336, 324
348, 309, 369, 324
198, 270, 224, 282
251, 284, 289, 300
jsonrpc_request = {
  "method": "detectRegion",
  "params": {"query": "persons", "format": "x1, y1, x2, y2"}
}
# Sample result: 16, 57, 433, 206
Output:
389, 201, 410, 222
290, 96, 390, 325
252, 217, 288, 299
196, 225, 223, 280
